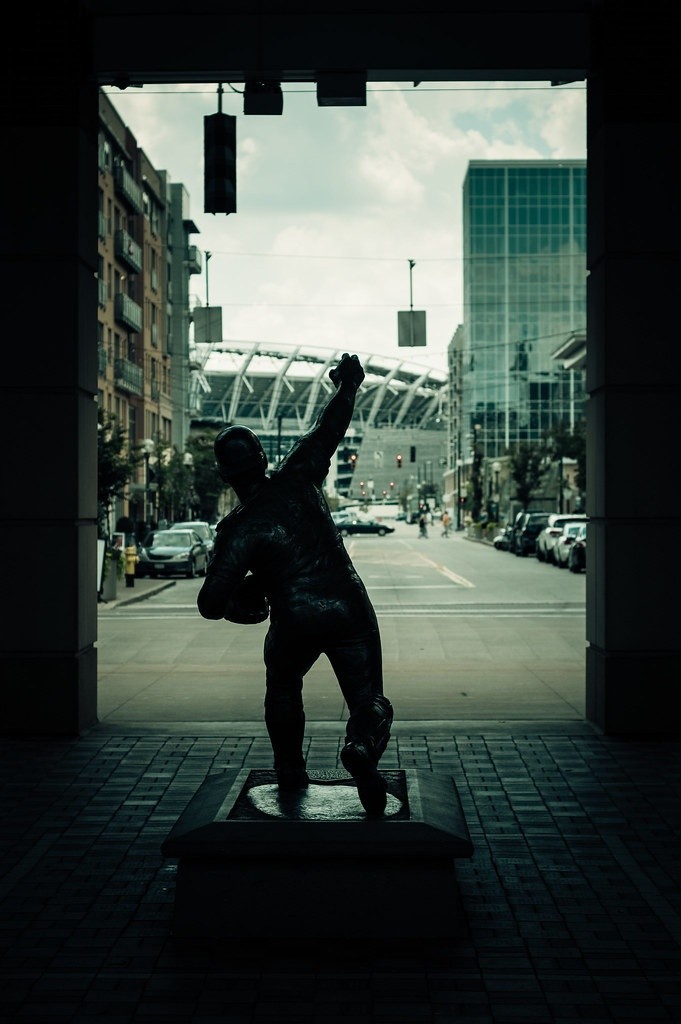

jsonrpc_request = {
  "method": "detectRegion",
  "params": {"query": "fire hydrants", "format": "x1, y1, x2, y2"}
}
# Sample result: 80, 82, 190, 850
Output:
123, 546, 141, 588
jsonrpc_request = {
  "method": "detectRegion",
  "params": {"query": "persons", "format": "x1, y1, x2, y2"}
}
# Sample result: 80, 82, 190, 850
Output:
440, 511, 452, 539
480, 509, 489, 529
417, 514, 428, 539
198, 353, 394, 814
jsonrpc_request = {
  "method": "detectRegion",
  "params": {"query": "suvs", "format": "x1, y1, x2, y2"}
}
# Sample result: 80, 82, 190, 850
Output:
536, 513, 586, 565
167, 521, 216, 563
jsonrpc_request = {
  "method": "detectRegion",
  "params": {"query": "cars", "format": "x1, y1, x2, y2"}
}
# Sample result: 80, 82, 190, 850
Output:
396, 512, 407, 521
336, 514, 396, 537
134, 529, 210, 579
550, 522, 586, 567
494, 509, 552, 557
331, 511, 356, 526
566, 524, 586, 573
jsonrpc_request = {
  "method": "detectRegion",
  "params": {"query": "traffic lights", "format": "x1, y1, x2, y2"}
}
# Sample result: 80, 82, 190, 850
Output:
383, 490, 387, 495
459, 497, 465, 503
362, 491, 366, 496
360, 481, 364, 487
351, 450, 358, 463
390, 482, 394, 488
419, 503, 424, 510
397, 454, 403, 468
